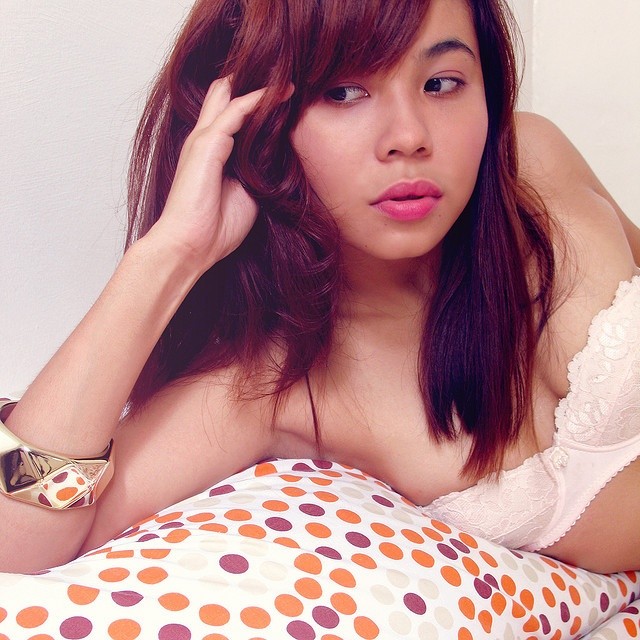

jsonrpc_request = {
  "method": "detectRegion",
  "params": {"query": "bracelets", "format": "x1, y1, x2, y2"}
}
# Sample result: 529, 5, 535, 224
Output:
0, 398, 115, 514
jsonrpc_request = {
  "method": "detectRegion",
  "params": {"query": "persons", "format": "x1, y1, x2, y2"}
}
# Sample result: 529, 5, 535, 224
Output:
0, 1, 638, 575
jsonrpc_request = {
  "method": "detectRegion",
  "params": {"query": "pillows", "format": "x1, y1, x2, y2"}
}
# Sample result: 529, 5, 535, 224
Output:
0, 458, 639, 640
